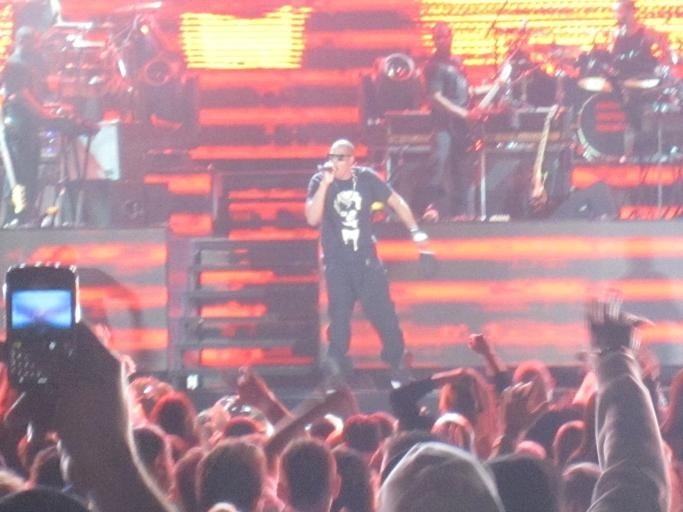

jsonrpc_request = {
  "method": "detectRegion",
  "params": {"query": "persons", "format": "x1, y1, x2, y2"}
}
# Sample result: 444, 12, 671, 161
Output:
304, 138, 430, 369
604, 1, 678, 70
423, 20, 481, 225
633, 339, 681, 512
581, 296, 655, 512
1, 322, 166, 507
4, 23, 74, 230
93, 322, 595, 511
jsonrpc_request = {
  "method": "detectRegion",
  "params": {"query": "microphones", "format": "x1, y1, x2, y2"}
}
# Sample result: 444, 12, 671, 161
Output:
316, 165, 332, 172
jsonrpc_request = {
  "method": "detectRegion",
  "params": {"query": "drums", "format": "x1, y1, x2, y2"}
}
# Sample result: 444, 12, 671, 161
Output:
575, 91, 632, 166
577, 52, 620, 92
622, 52, 662, 88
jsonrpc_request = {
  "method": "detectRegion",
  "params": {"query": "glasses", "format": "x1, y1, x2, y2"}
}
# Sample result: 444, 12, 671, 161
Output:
327, 153, 350, 161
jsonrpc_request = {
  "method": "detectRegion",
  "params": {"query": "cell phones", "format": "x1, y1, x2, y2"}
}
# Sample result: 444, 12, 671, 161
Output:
4, 264, 79, 393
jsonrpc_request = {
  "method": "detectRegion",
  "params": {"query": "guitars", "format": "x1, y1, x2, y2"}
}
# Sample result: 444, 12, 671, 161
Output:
516, 97, 562, 218
